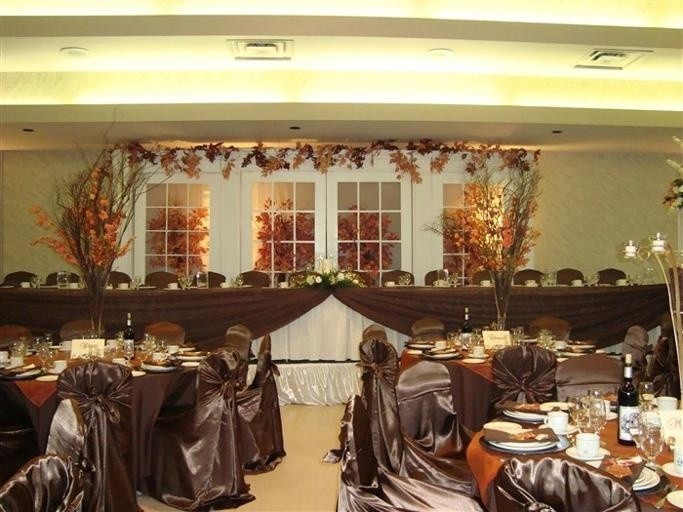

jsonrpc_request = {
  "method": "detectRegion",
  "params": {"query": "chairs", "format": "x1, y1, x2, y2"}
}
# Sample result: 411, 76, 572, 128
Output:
234, 333, 286, 475
320, 394, 483, 512
108, 271, 132, 289
625, 246, 636, 256
555, 355, 624, 403
653, 240, 664, 251
487, 456, 642, 512
528, 316, 569, 341
411, 318, 444, 341
622, 325, 649, 368
60, 319, 99, 341
513, 269, 544, 286
0, 455, 85, 512
145, 272, 181, 289
381, 270, 414, 287
355, 338, 477, 496
162, 351, 256, 512
288, 271, 321, 287
342, 271, 370, 287
4, 271, 38, 288
486, 345, 558, 423
225, 324, 252, 386
44, 399, 97, 512
596, 268, 626, 287
240, 271, 270, 288
640, 336, 673, 397
425, 269, 447, 286
363, 324, 388, 342
0, 326, 31, 345
145, 320, 186, 345
57, 361, 136, 512
472, 270, 492, 285
395, 361, 475, 461
192, 272, 226, 287
46, 271, 80, 287
557, 269, 584, 285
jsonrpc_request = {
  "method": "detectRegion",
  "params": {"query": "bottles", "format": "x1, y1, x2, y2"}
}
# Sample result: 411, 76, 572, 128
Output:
617, 353, 639, 446
123, 312, 135, 361
461, 307, 473, 338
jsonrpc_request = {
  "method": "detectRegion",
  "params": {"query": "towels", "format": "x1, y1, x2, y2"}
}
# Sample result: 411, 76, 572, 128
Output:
597, 455, 648, 486
0, 362, 42, 377
483, 427, 560, 443
494, 400, 560, 416
556, 346, 591, 354
142, 359, 183, 367
422, 349, 458, 356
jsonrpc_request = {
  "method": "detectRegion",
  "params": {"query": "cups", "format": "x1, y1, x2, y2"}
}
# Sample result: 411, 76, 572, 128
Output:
473, 346, 484, 357
605, 400, 613, 418
544, 411, 566, 433
576, 433, 600, 458
637, 413, 663, 470
577, 410, 589, 433
658, 396, 677, 412
673, 449, 683, 474
638, 382, 654, 411
590, 400, 606, 435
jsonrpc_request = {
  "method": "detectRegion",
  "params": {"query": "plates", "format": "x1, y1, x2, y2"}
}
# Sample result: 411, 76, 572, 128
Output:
468, 354, 490, 358
463, 358, 479, 360
488, 439, 557, 451
503, 409, 544, 421
632, 466, 661, 491
662, 462, 683, 478
666, 490, 683, 508
501, 421, 544, 425
539, 424, 578, 436
565, 446, 611, 462
479, 434, 570, 455
540, 402, 575, 411
606, 412, 618, 420
428, 354, 456, 359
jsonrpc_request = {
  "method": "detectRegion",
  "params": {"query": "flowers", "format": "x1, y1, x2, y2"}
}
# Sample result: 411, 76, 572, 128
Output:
293, 265, 368, 288
